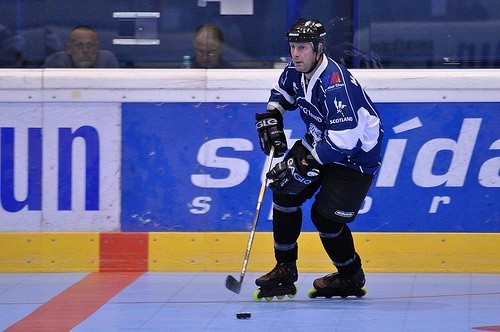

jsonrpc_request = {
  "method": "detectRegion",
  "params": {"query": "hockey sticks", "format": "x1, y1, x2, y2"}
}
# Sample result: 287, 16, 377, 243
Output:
224, 145, 276, 295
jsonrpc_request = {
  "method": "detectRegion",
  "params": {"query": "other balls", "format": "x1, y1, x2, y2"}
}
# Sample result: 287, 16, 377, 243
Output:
236, 311, 252, 320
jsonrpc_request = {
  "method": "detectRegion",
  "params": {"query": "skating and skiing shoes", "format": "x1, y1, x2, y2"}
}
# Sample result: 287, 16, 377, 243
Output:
308, 251, 367, 298
252, 242, 298, 301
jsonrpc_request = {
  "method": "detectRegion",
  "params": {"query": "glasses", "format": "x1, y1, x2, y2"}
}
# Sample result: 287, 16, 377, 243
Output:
193, 46, 218, 56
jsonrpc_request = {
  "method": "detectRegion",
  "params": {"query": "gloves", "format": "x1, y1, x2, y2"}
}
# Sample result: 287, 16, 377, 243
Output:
266, 153, 320, 195
255, 111, 287, 157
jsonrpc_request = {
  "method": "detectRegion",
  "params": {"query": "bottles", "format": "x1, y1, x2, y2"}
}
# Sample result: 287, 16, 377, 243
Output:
180, 55, 193, 68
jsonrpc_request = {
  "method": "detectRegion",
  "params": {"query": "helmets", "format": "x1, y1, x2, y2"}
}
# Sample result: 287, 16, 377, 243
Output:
285, 17, 327, 51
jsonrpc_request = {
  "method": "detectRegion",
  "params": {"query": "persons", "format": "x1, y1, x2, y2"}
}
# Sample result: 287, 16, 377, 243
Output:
43, 26, 120, 68
255, 17, 384, 291
181, 22, 235, 69
